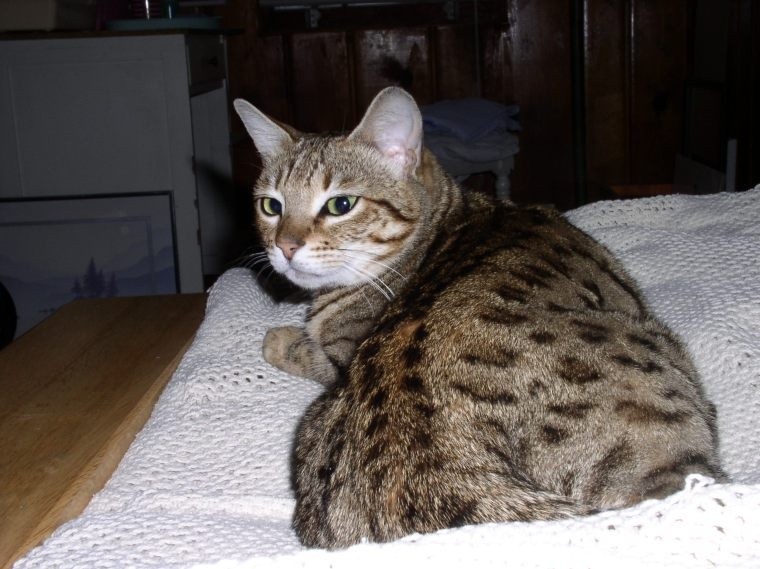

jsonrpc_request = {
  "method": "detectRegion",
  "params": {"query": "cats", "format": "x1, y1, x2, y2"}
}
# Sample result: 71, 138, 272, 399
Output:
233, 87, 734, 552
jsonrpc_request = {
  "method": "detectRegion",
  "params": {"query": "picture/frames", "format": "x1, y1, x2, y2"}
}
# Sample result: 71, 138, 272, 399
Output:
0, 190, 181, 352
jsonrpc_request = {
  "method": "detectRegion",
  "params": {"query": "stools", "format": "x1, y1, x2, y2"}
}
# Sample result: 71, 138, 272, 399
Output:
428, 138, 514, 201
